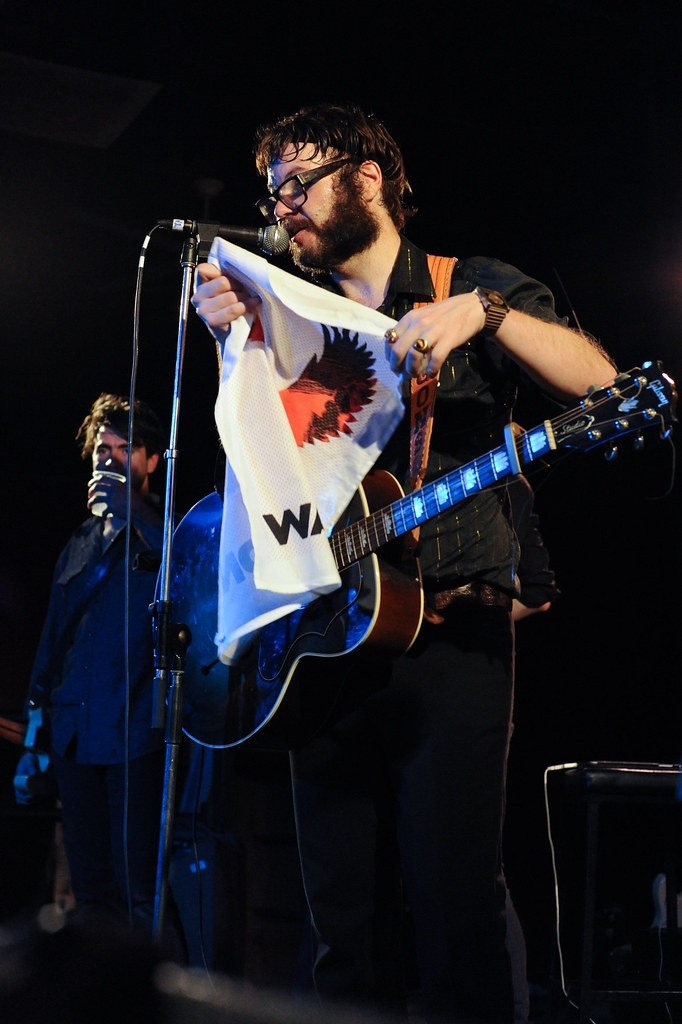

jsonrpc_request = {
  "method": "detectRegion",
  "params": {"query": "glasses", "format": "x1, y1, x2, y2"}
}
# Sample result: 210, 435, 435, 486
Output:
255, 158, 353, 223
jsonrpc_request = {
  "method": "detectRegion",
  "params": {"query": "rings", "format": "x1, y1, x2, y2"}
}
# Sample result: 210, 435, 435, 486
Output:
381, 328, 399, 344
412, 337, 430, 355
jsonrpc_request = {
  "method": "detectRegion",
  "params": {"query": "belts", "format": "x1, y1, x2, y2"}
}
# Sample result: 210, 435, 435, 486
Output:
432, 577, 516, 611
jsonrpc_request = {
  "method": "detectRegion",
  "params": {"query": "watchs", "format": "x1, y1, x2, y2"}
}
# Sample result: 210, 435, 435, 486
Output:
475, 285, 510, 336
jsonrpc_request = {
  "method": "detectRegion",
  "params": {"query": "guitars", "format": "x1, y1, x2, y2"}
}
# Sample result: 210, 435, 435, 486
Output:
141, 352, 681, 761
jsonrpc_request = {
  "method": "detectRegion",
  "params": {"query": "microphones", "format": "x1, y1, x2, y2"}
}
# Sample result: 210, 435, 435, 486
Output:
158, 220, 289, 256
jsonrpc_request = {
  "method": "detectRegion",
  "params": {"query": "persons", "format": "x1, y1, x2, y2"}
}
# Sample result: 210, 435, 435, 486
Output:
24, 391, 190, 970
192, 104, 621, 1024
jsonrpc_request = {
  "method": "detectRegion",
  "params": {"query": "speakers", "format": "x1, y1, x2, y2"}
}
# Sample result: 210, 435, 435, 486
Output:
542, 767, 682, 991
544, 992, 682, 1024
